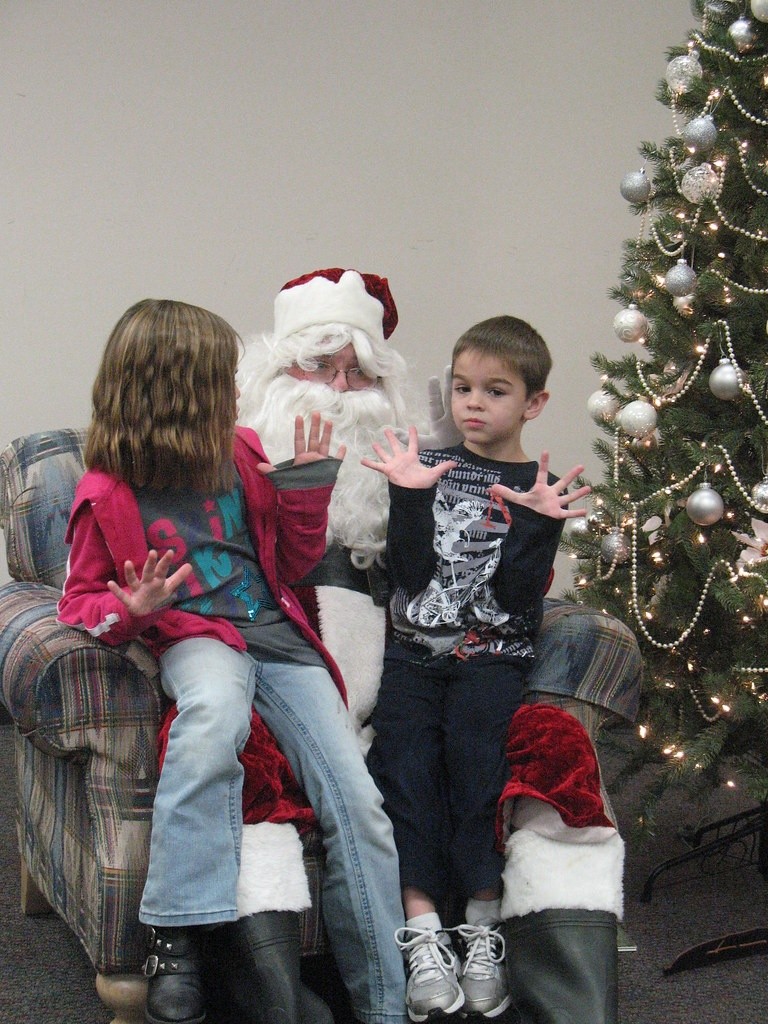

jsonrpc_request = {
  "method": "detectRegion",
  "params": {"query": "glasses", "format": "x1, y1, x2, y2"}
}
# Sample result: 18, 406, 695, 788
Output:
303, 361, 379, 389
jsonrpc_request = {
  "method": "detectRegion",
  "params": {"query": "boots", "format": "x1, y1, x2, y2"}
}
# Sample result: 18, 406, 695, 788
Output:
212, 822, 313, 1024
500, 824, 625, 1024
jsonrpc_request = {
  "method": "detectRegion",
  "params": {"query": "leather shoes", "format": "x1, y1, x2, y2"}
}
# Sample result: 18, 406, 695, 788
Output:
142, 923, 205, 1024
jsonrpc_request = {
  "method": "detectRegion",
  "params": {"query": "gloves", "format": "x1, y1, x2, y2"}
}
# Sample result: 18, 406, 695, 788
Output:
395, 365, 465, 450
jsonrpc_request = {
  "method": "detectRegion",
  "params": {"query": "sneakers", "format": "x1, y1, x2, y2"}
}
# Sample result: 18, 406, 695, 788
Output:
457, 916, 511, 1020
394, 924, 464, 1023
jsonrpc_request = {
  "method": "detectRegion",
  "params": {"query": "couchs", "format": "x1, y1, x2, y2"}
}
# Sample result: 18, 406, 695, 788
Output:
0, 431, 644, 1024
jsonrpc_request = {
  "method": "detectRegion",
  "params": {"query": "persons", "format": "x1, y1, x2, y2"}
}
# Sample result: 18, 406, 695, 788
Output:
359, 315, 593, 1024
57, 299, 408, 1024
161, 264, 628, 1024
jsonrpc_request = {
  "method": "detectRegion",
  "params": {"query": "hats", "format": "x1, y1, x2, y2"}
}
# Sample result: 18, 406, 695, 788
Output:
273, 267, 398, 345
